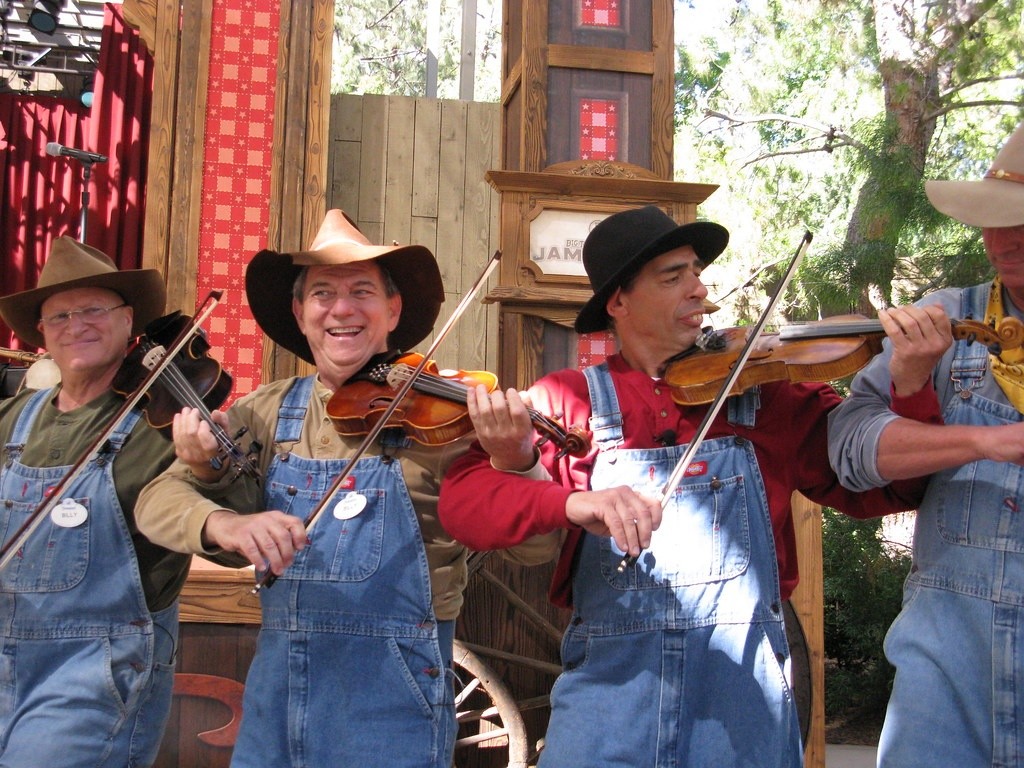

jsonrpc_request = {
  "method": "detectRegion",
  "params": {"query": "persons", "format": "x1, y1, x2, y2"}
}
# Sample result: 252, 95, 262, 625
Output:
133, 208, 567, 768
827, 125, 1024, 768
438, 204, 957, 768
1, 236, 260, 768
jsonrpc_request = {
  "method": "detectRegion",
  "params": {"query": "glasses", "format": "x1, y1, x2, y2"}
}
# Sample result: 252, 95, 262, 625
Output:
39, 303, 124, 333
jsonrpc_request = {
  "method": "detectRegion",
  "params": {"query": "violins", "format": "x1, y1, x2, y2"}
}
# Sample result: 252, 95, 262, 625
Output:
664, 312, 1024, 407
325, 346, 595, 462
108, 308, 265, 485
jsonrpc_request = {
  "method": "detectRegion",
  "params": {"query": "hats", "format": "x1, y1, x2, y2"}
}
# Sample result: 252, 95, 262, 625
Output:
925, 121, 1024, 228
0, 234, 167, 351
573, 205, 729, 333
245, 208, 445, 366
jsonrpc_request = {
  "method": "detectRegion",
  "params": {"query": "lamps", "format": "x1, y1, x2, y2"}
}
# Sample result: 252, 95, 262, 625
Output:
26, 0, 63, 37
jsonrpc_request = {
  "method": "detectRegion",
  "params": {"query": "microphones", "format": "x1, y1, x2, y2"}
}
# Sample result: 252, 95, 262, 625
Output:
45, 142, 109, 163
